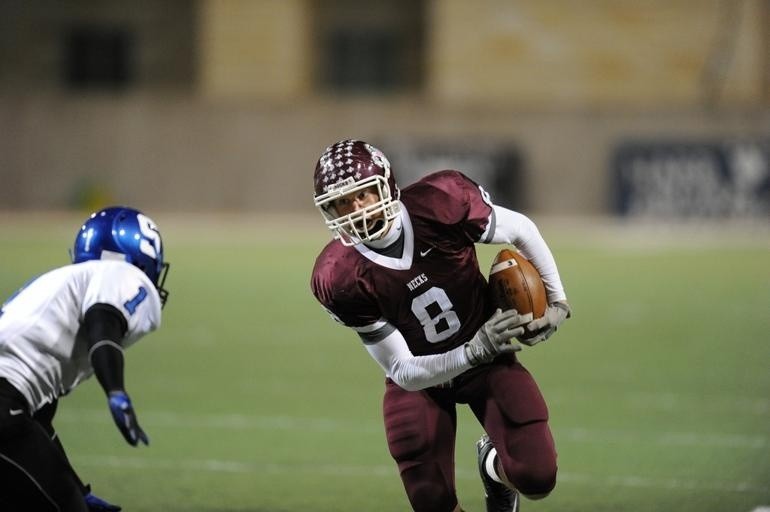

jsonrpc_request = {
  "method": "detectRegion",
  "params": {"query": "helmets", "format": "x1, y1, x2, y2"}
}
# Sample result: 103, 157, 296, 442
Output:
72, 203, 165, 286
307, 133, 402, 213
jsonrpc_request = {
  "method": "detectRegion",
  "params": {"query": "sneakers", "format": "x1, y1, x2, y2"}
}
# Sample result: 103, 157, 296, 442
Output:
475, 432, 521, 511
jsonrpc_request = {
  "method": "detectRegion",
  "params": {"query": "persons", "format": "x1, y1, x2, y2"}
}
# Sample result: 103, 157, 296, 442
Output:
309, 138, 573, 510
0, 202, 170, 510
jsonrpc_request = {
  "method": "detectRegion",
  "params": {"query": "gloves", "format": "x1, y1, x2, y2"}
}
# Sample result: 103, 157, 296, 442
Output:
105, 388, 151, 449
462, 305, 526, 366
517, 302, 572, 348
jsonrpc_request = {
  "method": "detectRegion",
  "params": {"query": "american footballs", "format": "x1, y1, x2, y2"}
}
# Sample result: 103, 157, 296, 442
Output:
488, 248, 547, 339
